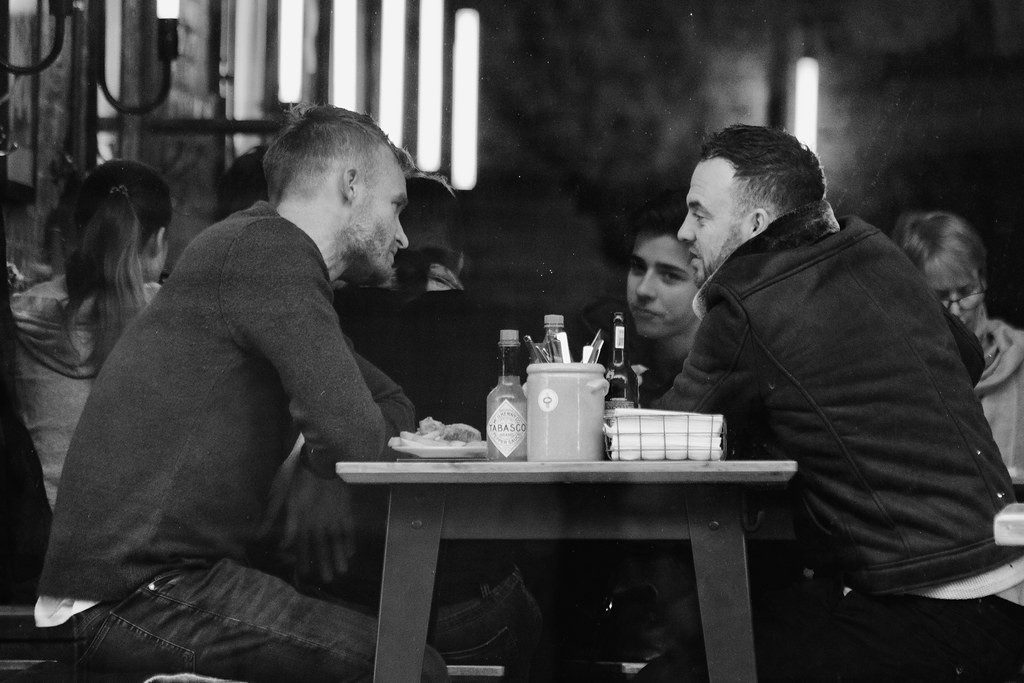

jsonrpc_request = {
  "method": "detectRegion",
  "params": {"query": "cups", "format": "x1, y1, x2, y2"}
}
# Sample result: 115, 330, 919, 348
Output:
521, 361, 610, 462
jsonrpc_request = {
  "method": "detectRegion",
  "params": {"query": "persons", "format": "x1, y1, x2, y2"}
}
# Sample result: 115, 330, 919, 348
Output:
0, 125, 1024, 683
34, 105, 450, 683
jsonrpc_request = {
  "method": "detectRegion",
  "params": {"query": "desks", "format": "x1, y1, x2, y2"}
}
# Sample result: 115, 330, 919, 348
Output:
335, 460, 798, 683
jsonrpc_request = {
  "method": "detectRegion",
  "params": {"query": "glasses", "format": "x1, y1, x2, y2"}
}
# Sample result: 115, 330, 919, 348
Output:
940, 278, 988, 310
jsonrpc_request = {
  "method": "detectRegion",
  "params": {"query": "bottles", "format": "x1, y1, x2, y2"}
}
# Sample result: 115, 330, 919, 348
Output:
603, 311, 637, 415
542, 314, 568, 366
485, 327, 529, 464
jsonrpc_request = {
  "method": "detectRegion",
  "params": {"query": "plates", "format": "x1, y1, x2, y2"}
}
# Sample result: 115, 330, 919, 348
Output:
391, 446, 487, 459
